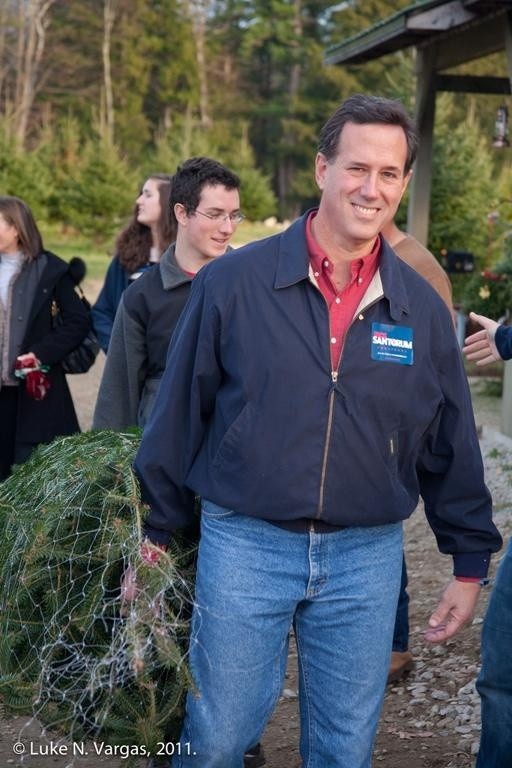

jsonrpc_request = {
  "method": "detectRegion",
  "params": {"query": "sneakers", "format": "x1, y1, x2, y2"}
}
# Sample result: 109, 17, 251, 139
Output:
386, 650, 415, 682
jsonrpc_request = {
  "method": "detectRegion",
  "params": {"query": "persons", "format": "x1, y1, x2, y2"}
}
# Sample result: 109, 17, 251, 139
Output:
113, 93, 505, 766
462, 306, 510, 766
0, 195, 90, 481
91, 156, 268, 767
374, 214, 456, 685
92, 175, 175, 356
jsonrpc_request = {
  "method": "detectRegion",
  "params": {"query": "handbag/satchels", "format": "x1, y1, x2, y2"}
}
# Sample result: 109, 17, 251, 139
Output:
52, 296, 100, 374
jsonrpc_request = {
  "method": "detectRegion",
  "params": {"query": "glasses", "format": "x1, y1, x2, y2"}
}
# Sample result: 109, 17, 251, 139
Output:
182, 203, 246, 225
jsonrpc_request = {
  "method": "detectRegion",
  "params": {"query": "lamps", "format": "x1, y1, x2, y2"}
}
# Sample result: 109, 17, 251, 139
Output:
491, 93, 510, 150
